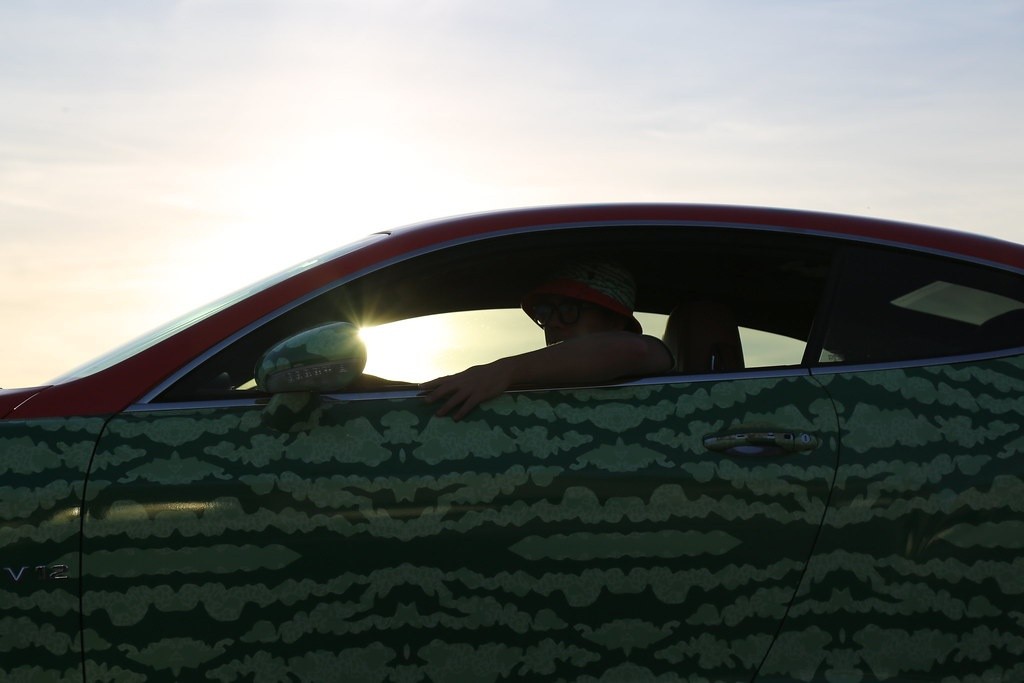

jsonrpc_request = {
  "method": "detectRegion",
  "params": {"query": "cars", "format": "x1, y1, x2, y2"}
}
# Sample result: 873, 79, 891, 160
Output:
0, 203, 1024, 683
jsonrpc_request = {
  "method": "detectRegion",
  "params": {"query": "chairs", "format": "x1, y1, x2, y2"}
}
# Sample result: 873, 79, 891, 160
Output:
661, 288, 744, 377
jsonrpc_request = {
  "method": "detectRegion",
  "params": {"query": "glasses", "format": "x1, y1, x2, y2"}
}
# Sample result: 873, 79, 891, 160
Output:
529, 301, 582, 327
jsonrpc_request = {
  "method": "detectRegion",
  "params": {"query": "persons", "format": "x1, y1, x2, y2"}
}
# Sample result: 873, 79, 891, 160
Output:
415, 245, 685, 423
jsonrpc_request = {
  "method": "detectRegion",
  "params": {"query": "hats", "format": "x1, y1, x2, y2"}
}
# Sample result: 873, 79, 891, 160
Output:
520, 253, 643, 337
